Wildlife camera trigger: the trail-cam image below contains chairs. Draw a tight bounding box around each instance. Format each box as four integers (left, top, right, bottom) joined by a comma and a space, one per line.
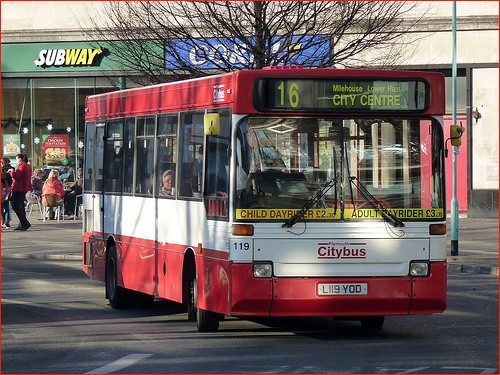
24, 193, 42, 221
41, 193, 65, 221
197, 156, 229, 194
73, 194, 83, 221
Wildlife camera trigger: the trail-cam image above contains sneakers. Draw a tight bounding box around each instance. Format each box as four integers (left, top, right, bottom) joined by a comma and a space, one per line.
44, 212, 77, 221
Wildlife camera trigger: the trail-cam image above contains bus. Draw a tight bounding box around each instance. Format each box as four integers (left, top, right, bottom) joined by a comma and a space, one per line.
81, 65, 465, 331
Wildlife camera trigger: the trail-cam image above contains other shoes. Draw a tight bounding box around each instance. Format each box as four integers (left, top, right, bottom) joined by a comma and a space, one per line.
22, 225, 31, 230
14, 225, 21, 231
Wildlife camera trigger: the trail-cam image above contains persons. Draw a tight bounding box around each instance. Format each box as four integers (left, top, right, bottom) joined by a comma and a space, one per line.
86, 110, 315, 225
29, 169, 55, 219
6, 154, 32, 231
63, 169, 82, 219
42, 170, 64, 219
1, 164, 12, 228
1, 158, 15, 229
